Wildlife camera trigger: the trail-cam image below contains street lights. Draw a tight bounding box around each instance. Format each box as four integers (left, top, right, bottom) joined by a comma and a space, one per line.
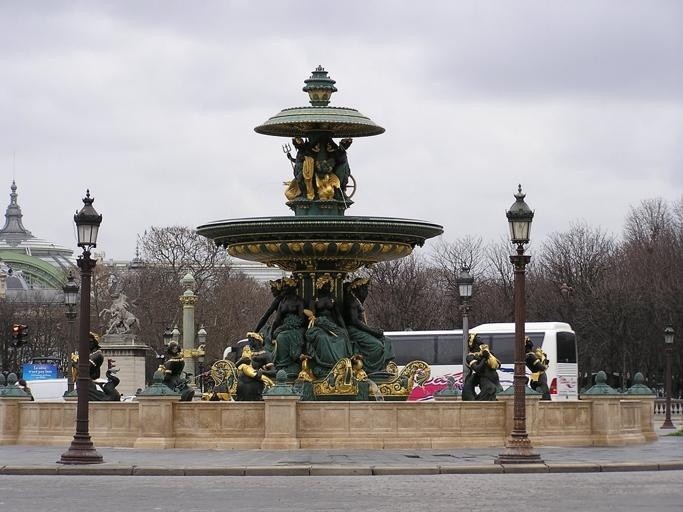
659, 322, 679, 429
198, 324, 206, 397
161, 323, 182, 352
456, 262, 475, 388
495, 185, 547, 463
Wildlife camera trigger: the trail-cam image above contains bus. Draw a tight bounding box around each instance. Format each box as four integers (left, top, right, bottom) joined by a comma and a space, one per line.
380, 322, 578, 400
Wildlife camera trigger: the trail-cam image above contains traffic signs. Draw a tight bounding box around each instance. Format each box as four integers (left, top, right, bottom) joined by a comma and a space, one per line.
56, 189, 105, 464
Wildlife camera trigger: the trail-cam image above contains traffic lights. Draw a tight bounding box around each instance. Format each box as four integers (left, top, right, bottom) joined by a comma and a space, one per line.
12, 323, 27, 348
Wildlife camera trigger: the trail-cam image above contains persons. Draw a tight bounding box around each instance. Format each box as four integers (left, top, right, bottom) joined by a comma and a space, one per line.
524, 335, 553, 400
283, 135, 311, 200
302, 137, 341, 201
159, 341, 185, 389
246, 273, 395, 379
464, 334, 502, 400
231, 331, 276, 400
331, 137, 352, 202
66, 331, 104, 381
